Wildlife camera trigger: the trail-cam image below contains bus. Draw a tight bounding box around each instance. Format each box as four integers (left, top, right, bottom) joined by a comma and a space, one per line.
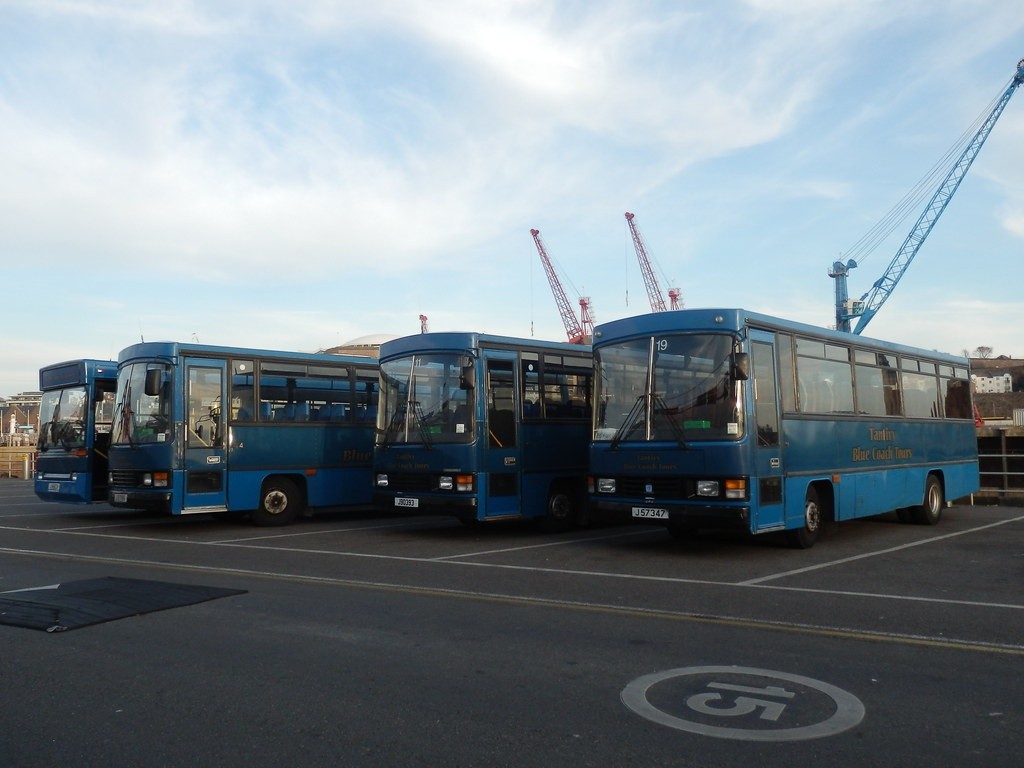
583, 307, 980, 550
370, 332, 770, 533
34, 359, 221, 506
106, 342, 465, 529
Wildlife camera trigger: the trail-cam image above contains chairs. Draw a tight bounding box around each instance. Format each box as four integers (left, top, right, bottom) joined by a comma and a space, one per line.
454, 396, 592, 420
784, 380, 945, 414
237, 401, 378, 423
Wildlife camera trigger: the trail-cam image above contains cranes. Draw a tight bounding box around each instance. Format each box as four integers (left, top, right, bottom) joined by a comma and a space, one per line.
825, 57, 1024, 338
623, 212, 685, 313
529, 228, 597, 347
